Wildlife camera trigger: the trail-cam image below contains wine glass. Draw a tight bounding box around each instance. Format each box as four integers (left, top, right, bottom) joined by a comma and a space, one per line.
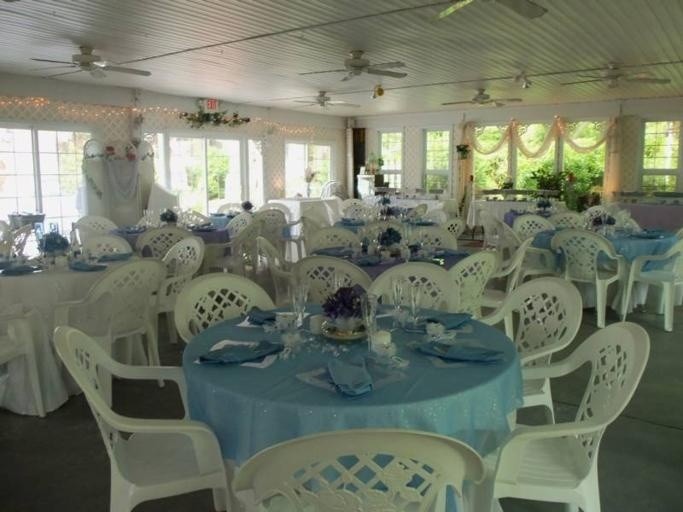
353, 192, 425, 263
389, 274, 424, 330
31, 221, 62, 263
575, 205, 633, 239
357, 292, 381, 357
287, 279, 311, 332
140, 204, 195, 232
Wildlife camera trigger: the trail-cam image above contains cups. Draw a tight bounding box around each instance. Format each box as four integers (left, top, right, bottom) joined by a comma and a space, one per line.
310, 314, 328, 335
376, 330, 392, 345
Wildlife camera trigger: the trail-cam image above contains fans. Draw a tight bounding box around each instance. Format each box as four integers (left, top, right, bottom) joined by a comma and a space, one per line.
559, 61, 671, 88
440, 88, 523, 108
30, 47, 151, 79
431, 0, 548, 24
298, 51, 408, 81
294, 91, 361, 111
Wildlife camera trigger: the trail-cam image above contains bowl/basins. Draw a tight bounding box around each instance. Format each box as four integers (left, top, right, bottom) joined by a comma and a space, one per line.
7, 213, 46, 232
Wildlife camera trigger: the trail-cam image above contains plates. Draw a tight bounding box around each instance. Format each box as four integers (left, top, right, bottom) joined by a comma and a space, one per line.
320, 318, 367, 341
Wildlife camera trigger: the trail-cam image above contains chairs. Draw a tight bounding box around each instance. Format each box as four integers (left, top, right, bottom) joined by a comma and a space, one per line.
483, 321, 651, 512
230, 428, 487, 512
1, 215, 133, 420
170, 250, 586, 428
298, 199, 468, 263
478, 200, 680, 332
52, 326, 228, 512
135, 205, 293, 305
54, 256, 170, 391
